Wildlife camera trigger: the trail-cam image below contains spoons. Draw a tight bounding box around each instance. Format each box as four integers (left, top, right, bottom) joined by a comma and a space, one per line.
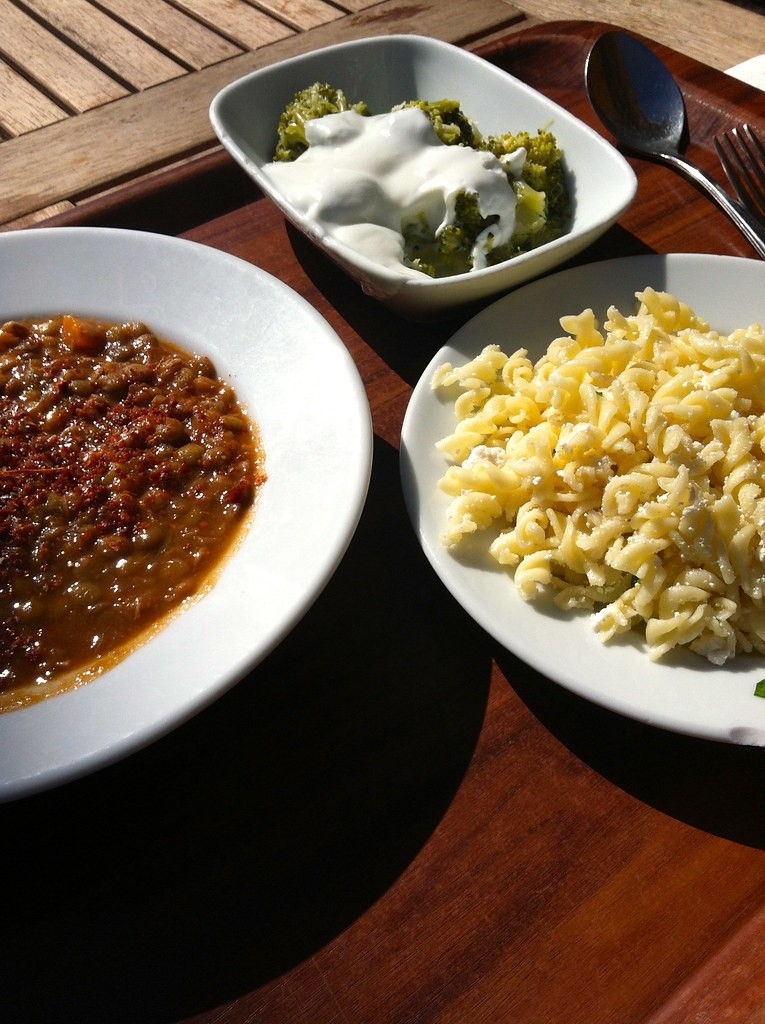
586, 30, 765, 264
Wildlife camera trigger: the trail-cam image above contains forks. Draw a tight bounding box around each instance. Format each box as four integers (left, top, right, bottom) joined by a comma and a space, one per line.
709, 122, 765, 239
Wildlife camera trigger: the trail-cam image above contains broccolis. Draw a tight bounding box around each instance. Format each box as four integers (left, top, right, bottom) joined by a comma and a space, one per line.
273, 81, 567, 277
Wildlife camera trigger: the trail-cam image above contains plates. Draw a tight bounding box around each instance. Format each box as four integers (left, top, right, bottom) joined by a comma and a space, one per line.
209, 35, 638, 318
0, 228, 374, 794
396, 253, 765, 747
